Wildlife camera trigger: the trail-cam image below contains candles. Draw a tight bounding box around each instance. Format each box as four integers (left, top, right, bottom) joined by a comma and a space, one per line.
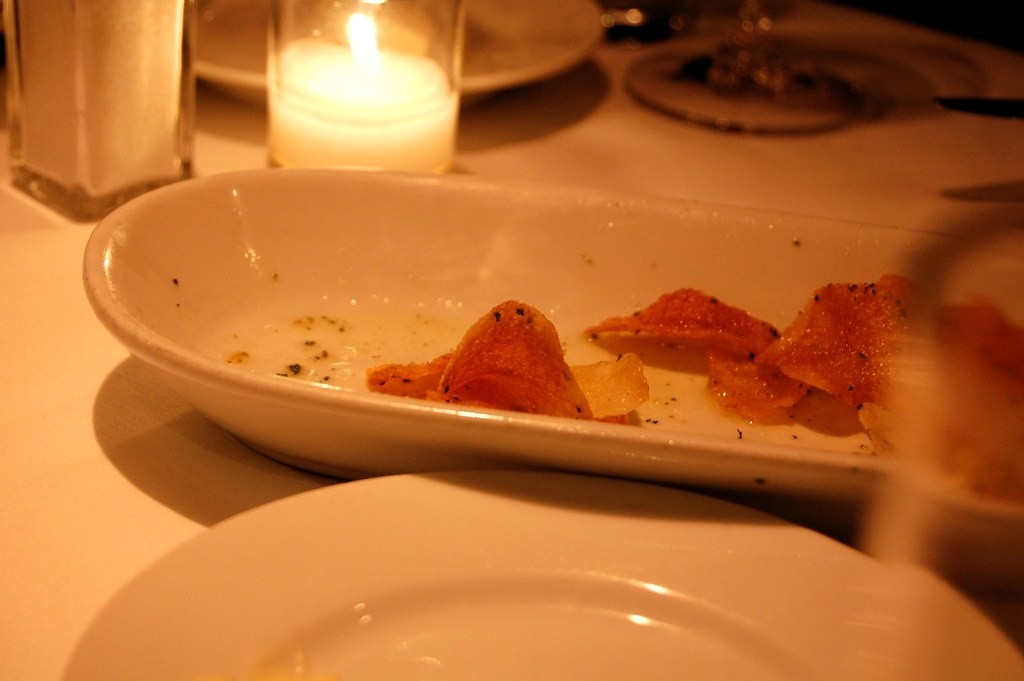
267, 11, 451, 174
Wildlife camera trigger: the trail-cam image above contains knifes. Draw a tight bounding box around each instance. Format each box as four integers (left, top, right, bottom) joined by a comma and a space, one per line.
932, 96, 1024, 120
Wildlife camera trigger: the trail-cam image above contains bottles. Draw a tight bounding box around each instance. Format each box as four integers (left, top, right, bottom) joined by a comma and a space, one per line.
0, 1, 194, 222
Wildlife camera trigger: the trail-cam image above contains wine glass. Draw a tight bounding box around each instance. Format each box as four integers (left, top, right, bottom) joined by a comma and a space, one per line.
628, 0, 853, 133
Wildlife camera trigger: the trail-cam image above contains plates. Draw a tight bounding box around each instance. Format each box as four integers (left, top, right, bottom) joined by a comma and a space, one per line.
59, 473, 1024, 680
84, 170, 1024, 506
186, 1, 604, 95
